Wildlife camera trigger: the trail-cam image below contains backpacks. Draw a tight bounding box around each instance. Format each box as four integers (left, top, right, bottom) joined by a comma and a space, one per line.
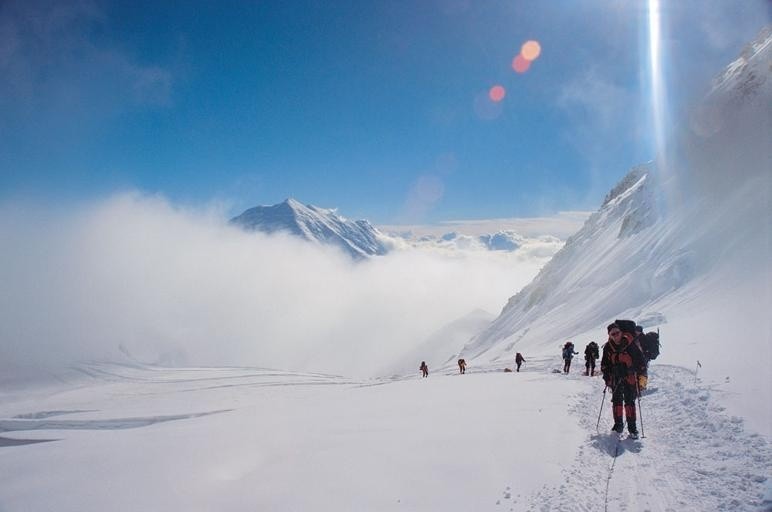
615, 319, 646, 375
644, 331, 659, 359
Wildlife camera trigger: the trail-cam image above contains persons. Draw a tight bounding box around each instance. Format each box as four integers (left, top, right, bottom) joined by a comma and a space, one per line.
516, 353, 525, 372
633, 326, 650, 397
421, 362, 428, 377
601, 324, 646, 438
458, 359, 466, 374
585, 342, 600, 376
563, 342, 579, 373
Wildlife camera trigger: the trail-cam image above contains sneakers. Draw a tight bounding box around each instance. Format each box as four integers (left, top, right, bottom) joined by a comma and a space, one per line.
628, 424, 638, 433
615, 423, 623, 433
